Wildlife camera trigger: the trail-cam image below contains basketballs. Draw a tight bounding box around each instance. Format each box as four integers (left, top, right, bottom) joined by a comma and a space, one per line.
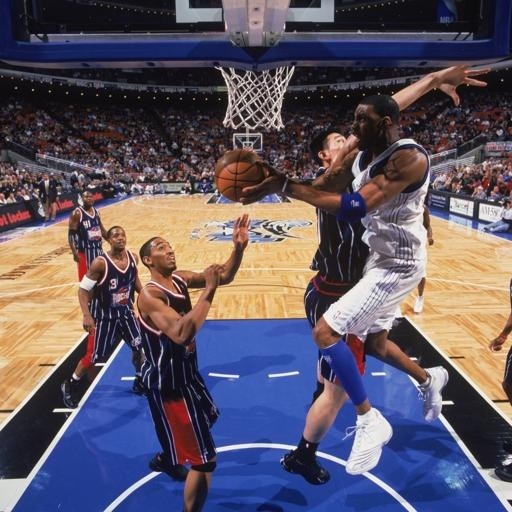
214, 150, 268, 202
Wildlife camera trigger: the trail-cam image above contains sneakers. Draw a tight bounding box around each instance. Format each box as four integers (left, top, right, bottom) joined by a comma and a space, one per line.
281, 450, 330, 485
414, 297, 424, 313
419, 366, 449, 421
495, 463, 512, 482
61, 379, 79, 408
132, 377, 147, 396
346, 409, 394, 476
150, 453, 189, 480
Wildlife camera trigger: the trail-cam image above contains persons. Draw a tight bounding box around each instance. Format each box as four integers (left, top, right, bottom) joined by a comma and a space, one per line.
489, 278, 512, 482
61, 225, 148, 407
239, 97, 450, 475
281, 63, 491, 485
2, 1, 512, 232
136, 213, 251, 511
68, 192, 110, 310
414, 202, 435, 313
